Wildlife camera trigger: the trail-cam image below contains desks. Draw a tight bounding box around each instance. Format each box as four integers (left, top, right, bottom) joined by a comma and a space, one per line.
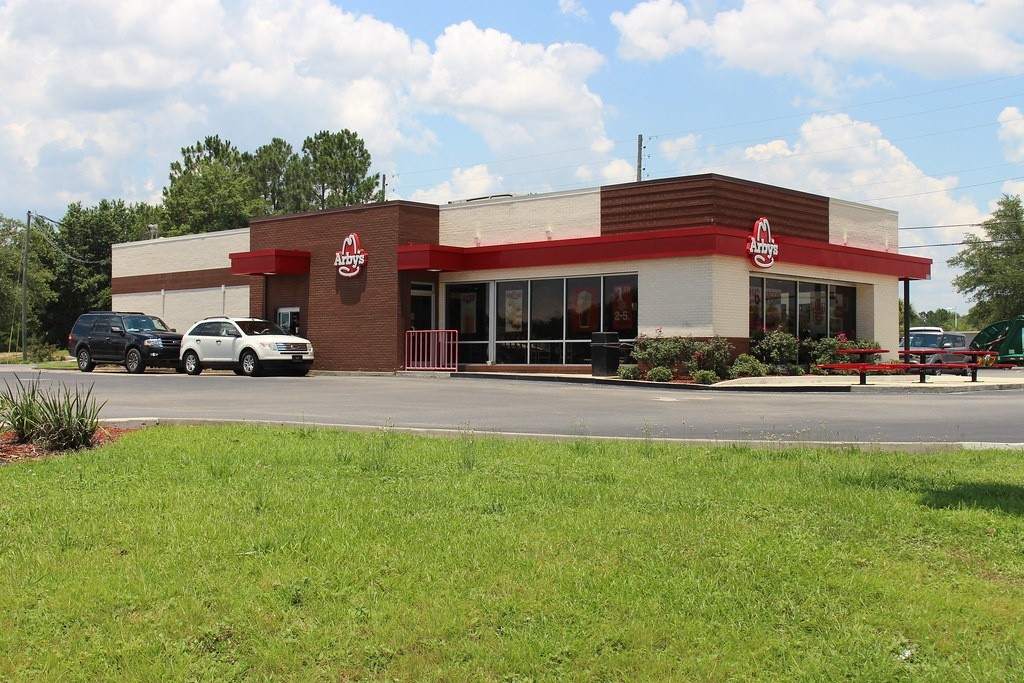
952, 351, 999, 382
896, 349, 946, 383
836, 349, 890, 385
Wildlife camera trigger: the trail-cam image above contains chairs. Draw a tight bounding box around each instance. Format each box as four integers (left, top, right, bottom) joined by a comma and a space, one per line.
524, 337, 576, 364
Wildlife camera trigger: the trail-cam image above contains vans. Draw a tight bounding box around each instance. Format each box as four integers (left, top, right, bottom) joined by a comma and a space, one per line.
899, 327, 945, 348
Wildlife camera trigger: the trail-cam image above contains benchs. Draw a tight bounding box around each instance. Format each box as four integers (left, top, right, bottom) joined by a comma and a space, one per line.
817, 364, 1015, 380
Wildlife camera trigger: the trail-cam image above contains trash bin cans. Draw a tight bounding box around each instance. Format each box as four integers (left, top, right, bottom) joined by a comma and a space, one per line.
590, 332, 620, 377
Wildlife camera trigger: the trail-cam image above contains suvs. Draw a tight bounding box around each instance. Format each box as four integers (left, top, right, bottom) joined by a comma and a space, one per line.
900, 332, 972, 377
178, 316, 315, 376
68, 311, 184, 374
